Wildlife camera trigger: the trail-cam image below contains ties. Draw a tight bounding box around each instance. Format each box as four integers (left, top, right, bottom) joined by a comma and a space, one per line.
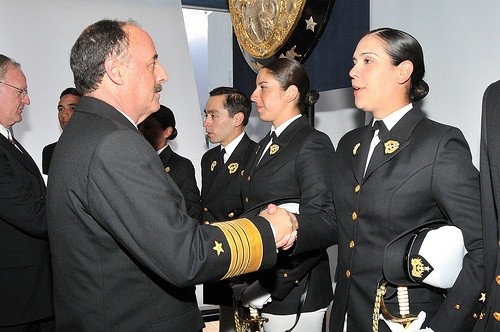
218, 148, 226, 169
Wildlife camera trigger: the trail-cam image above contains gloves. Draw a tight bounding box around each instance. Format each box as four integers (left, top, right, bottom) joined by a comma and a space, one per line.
233, 283, 272, 306
241, 279, 270, 310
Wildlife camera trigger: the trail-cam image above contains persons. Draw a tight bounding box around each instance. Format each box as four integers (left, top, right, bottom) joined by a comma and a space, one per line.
141, 103, 203, 227
41, 87, 82, 175
199, 55, 338, 332
259, 27, 500, 332
43, 18, 297, 332
0, 51, 56, 332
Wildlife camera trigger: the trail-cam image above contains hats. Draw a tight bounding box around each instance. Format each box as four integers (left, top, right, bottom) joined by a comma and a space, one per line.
238, 196, 301, 219
382, 219, 468, 288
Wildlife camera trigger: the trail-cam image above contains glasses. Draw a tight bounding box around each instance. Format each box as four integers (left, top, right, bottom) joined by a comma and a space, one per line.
0, 81, 28, 99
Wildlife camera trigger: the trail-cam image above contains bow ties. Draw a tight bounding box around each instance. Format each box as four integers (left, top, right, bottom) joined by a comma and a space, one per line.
270, 131, 278, 143
370, 120, 389, 140
